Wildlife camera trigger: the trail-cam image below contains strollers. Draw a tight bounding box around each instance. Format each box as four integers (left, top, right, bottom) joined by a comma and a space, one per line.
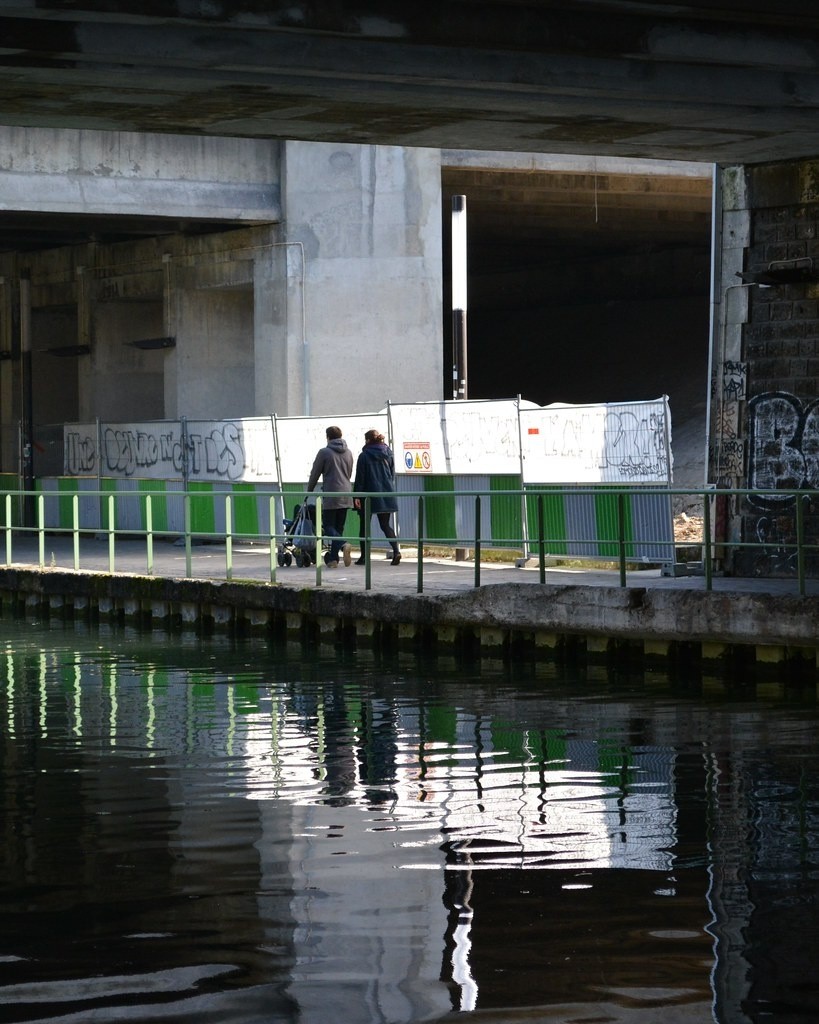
275, 496, 341, 569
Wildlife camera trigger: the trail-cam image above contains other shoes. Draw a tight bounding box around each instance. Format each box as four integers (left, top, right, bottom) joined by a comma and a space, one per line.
326, 561, 338, 568
341, 543, 351, 567
390, 553, 402, 566
355, 557, 365, 565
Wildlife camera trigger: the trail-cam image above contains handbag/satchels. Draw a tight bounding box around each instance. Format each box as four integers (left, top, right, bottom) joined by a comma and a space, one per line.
292, 501, 316, 551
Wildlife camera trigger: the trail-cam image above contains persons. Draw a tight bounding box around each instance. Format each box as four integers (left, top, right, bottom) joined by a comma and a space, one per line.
307, 426, 353, 568
352, 429, 402, 565
321, 671, 399, 808
